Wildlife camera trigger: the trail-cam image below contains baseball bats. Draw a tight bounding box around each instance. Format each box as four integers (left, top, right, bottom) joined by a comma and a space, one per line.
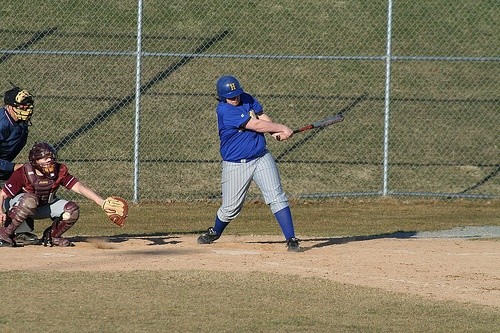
276, 114, 345, 141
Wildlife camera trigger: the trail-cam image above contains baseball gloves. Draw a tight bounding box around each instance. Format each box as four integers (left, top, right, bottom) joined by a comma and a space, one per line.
102, 195, 129, 227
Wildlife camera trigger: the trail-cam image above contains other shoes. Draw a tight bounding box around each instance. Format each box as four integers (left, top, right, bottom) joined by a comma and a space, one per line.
50, 237, 70, 246
8, 236, 24, 247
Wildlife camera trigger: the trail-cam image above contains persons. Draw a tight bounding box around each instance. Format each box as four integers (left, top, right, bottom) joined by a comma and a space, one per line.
197, 73, 300, 253
0, 143, 128, 247
0, 86, 34, 190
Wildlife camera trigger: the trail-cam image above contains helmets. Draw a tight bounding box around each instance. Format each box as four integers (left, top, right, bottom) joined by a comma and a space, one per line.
4, 88, 34, 109
217, 75, 243, 99
28, 142, 58, 180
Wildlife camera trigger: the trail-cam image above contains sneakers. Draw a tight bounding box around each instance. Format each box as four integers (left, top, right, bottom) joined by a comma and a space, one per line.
285, 237, 301, 252
197, 227, 221, 244
